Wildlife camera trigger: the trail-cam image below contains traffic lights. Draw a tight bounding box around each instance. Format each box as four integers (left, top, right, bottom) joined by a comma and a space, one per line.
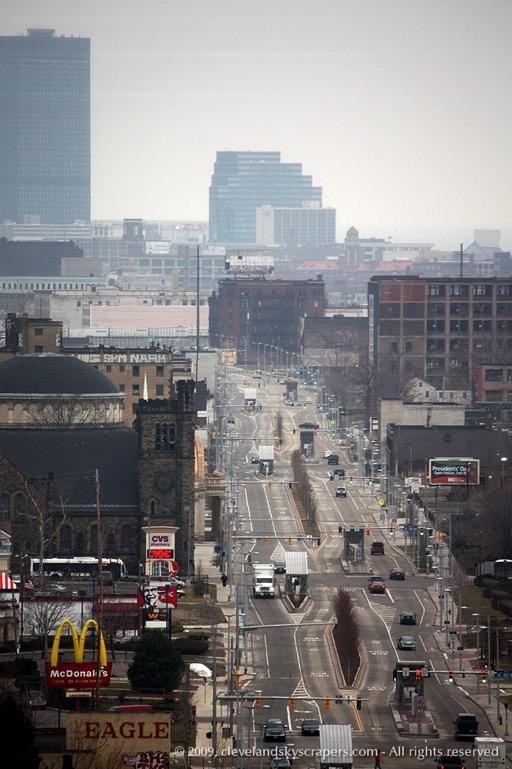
416, 669, 421, 681
393, 669, 397, 682
338, 527, 342, 536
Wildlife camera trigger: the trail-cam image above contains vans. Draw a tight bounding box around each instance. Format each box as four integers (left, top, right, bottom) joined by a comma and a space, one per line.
453, 712, 479, 741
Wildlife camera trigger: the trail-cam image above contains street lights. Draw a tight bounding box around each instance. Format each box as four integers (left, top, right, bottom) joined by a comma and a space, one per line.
363, 440, 421, 571
427, 535, 502, 720
309, 360, 352, 454
208, 376, 271, 769
215, 333, 306, 379
15, 552, 28, 652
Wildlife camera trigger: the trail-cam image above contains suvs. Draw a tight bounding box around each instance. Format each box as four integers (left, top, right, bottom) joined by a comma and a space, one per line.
28, 691, 47, 710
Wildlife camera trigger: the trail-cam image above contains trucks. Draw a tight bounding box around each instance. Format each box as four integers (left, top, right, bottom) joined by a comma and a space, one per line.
472, 737, 507, 768
320, 724, 353, 769
253, 563, 275, 599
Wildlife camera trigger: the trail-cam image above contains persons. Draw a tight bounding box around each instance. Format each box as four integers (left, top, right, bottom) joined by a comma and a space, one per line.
374, 748, 382, 769
137, 585, 177, 610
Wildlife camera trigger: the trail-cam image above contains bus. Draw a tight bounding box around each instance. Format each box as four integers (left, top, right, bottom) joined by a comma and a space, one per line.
30, 556, 127, 579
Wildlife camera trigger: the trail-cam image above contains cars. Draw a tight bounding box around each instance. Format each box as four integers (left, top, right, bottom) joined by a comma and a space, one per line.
270, 743, 295, 769
434, 756, 466, 769
368, 576, 386, 594
324, 450, 354, 498
389, 567, 405, 580
262, 719, 288, 742
140, 576, 186, 598
274, 561, 286, 573
399, 611, 417, 625
397, 635, 416, 650
302, 719, 321, 736
